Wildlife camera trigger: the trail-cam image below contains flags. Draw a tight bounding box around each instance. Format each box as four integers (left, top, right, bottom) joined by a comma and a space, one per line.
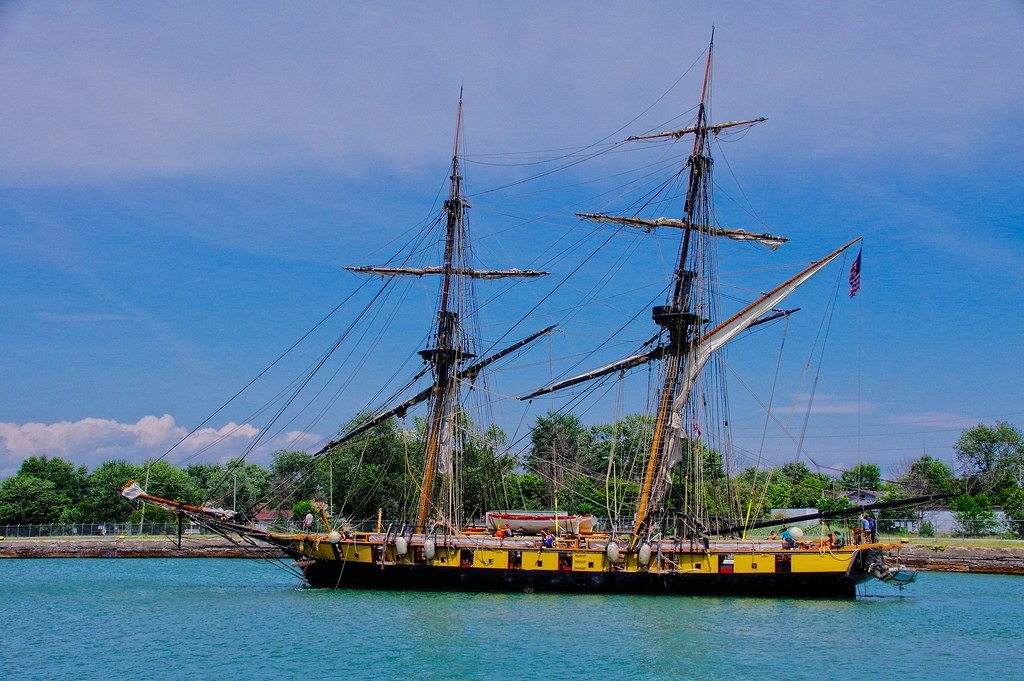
848, 249, 862, 298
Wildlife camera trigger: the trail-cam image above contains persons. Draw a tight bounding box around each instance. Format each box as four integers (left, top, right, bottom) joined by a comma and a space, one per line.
303, 510, 313, 532
767, 530, 779, 540
864, 509, 876, 543
780, 527, 796, 547
823, 534, 835, 545
834, 531, 843, 545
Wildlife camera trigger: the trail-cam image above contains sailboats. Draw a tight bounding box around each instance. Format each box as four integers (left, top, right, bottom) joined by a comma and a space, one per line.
118, 27, 917, 599
